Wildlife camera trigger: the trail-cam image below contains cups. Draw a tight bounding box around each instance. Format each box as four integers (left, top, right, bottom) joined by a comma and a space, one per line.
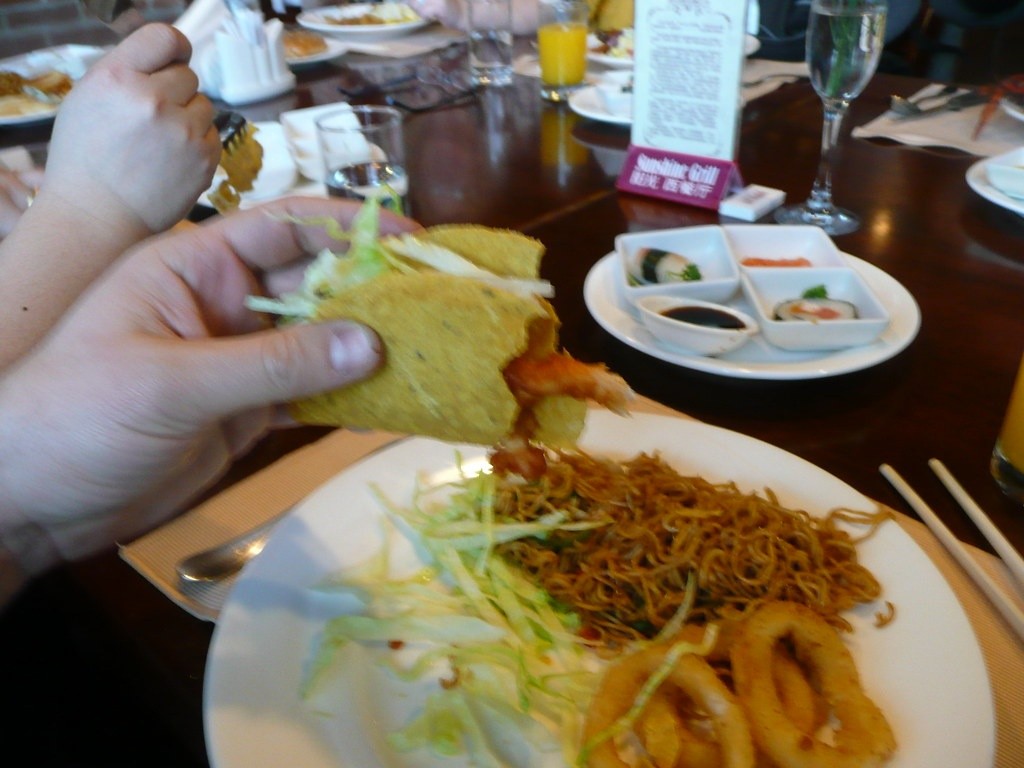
466, 1, 515, 89
537, 0, 588, 102
315, 104, 412, 219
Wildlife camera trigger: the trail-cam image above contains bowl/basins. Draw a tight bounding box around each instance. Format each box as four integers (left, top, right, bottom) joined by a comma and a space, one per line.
983, 147, 1024, 202
616, 221, 892, 355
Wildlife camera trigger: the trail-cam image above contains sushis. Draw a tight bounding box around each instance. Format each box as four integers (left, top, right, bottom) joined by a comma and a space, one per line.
631, 247, 700, 281
774, 296, 858, 320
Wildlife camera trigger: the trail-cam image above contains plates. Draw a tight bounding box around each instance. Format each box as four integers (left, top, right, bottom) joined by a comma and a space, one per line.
964, 154, 1024, 217
566, 85, 630, 126
586, 30, 761, 70
195, 120, 390, 208
284, 37, 349, 69
296, 2, 430, 44
584, 249, 920, 380
202, 406, 996, 768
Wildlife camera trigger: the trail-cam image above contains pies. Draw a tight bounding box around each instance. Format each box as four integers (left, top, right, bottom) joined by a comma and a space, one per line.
207, 119, 264, 213
245, 167, 636, 452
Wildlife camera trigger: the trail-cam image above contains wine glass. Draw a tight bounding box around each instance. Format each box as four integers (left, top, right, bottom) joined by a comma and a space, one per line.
774, 0, 888, 240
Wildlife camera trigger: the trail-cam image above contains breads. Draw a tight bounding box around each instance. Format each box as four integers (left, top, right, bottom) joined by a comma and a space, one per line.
284, 31, 326, 57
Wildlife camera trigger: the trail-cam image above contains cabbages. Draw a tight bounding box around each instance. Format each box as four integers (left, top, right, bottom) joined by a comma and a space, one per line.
298, 454, 718, 768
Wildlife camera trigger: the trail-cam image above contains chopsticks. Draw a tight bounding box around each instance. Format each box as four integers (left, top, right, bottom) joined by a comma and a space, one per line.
878, 459, 1024, 643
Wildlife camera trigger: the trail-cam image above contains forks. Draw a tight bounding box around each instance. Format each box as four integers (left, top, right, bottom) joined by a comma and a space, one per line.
211, 109, 249, 155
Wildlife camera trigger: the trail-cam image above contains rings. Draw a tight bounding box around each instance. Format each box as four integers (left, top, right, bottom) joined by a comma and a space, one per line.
417, 0, 425, 6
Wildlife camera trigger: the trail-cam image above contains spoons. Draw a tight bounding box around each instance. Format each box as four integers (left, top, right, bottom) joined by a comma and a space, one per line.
881, 88, 986, 117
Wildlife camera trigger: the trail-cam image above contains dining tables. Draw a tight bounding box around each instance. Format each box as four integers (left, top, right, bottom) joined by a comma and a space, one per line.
0, 0, 1023, 767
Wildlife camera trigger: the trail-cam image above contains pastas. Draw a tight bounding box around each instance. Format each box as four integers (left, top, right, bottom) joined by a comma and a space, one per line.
475, 450, 896, 651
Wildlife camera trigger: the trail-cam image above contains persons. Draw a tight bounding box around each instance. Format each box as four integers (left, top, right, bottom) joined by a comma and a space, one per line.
385, 0, 636, 44
0, 196, 430, 601
0, 23, 223, 376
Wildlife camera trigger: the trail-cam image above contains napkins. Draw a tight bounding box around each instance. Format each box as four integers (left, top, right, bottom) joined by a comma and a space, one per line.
852, 84, 1023, 158
738, 59, 810, 104
